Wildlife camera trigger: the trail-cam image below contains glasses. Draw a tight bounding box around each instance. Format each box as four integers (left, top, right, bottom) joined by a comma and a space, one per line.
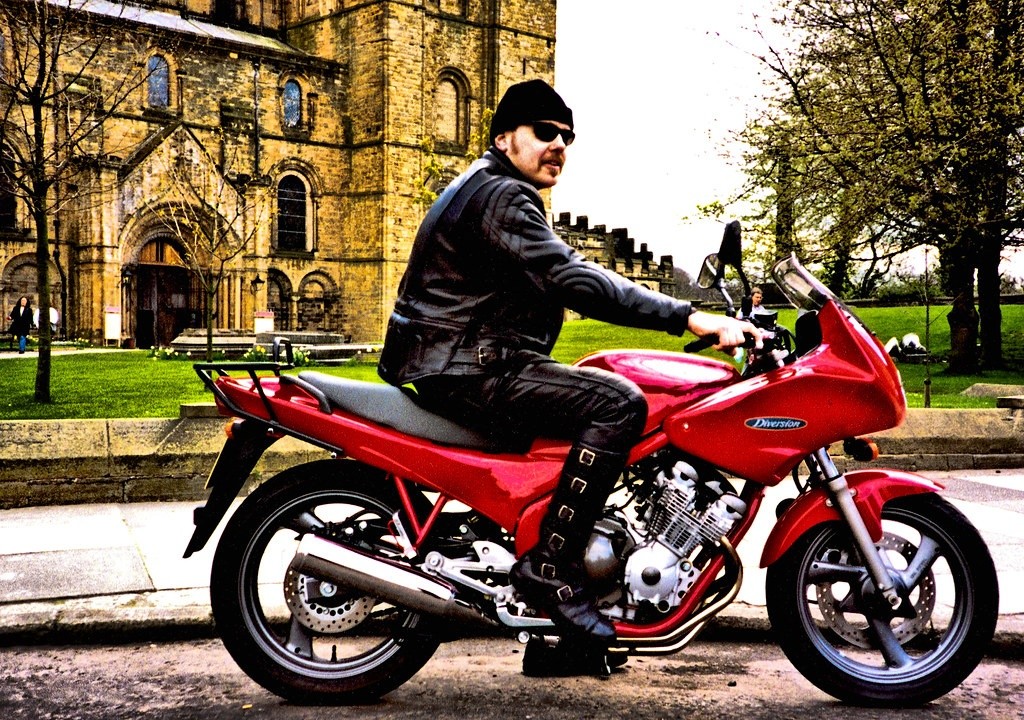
524, 118, 576, 146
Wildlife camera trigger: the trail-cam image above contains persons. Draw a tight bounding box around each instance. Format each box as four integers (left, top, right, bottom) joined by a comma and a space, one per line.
737, 287, 766, 320
376, 80, 765, 677
7, 297, 34, 354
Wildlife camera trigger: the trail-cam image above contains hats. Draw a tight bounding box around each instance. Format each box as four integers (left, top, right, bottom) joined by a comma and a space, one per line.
489, 79, 573, 142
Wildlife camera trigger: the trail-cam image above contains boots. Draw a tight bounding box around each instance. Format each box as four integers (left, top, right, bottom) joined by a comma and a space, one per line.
519, 630, 630, 677
509, 440, 633, 646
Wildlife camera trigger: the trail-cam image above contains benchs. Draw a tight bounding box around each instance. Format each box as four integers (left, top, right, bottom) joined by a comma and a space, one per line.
997, 395, 1024, 421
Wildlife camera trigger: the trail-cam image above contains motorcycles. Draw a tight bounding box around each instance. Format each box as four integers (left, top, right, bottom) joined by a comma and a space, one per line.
183, 220, 1000, 712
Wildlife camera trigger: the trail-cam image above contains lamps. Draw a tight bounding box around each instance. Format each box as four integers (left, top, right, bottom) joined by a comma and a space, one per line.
108, 155, 123, 164
249, 273, 265, 297
236, 173, 251, 184
116, 265, 133, 288
